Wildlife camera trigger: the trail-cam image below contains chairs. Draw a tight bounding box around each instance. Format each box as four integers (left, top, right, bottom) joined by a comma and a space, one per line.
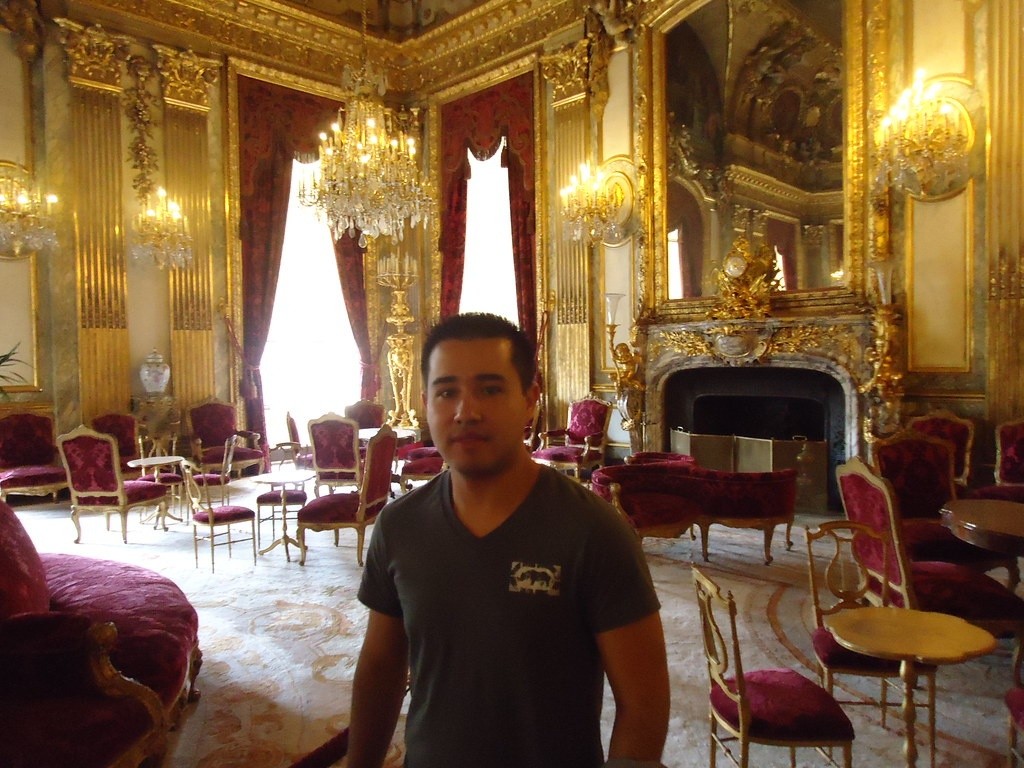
0, 396, 451, 574
522, 393, 613, 485
689, 410, 1024, 768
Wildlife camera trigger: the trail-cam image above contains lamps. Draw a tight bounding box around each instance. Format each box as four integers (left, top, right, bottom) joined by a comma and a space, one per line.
129, 185, 194, 270
0, 159, 63, 261
874, 69, 984, 201
296, 0, 435, 248
560, 156, 643, 248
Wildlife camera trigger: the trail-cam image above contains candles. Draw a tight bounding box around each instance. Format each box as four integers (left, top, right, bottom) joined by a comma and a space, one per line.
376, 246, 418, 277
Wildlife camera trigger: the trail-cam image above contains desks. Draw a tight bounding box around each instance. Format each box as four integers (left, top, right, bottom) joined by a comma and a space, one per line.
357, 428, 417, 475
248, 470, 316, 562
939, 498, 1024, 557
821, 607, 995, 768
126, 456, 185, 533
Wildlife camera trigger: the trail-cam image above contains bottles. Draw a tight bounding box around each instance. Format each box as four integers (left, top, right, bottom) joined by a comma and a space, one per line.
140, 348, 171, 399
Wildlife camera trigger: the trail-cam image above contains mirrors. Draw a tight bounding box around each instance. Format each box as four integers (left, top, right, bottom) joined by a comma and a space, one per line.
644, 0, 862, 323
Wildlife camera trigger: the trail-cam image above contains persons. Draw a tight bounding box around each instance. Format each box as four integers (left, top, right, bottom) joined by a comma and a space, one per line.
347, 313, 672, 767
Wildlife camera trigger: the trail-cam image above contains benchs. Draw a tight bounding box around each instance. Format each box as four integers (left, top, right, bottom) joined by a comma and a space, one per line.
0, 500, 203, 768
591, 465, 798, 567
